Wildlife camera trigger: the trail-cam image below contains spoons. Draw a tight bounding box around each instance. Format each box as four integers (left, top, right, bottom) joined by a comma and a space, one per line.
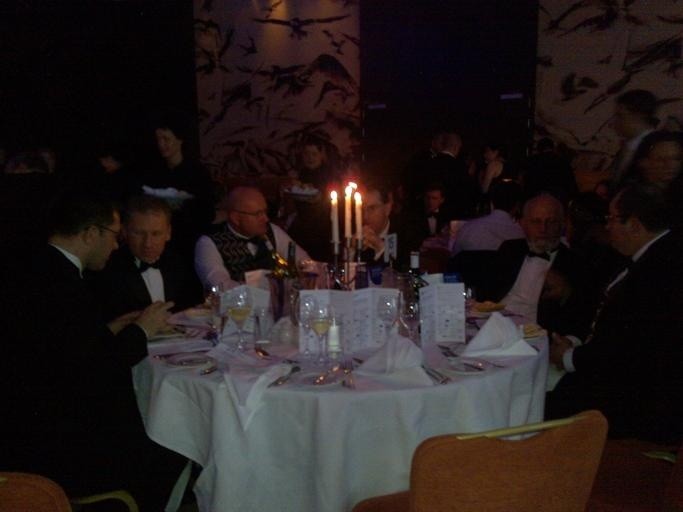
273, 364, 300, 386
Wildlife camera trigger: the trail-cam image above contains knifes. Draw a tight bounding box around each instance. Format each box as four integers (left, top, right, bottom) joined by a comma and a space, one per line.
152, 347, 211, 359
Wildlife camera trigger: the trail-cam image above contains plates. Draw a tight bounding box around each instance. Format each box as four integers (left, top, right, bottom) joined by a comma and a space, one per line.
145, 194, 190, 208
280, 191, 317, 202
182, 309, 211, 320
288, 367, 344, 389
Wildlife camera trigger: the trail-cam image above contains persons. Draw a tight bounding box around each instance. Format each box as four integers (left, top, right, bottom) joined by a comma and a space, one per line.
344, 172, 416, 274
398, 87, 681, 443
134, 122, 216, 215
0, 188, 191, 510
98, 193, 206, 329
193, 181, 316, 298
274, 137, 334, 250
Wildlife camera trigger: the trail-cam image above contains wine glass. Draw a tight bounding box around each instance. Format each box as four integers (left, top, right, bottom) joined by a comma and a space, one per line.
377, 295, 420, 346
223, 287, 251, 350
294, 295, 331, 367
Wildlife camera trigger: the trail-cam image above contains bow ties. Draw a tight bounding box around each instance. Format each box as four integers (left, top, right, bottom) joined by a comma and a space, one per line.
139, 261, 159, 271
528, 251, 550, 261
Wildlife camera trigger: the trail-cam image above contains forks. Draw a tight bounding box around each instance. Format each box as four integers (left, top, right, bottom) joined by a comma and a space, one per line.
344, 359, 354, 391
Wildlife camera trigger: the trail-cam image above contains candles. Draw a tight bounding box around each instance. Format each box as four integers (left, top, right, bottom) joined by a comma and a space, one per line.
344, 185, 352, 238
329, 190, 339, 242
355, 195, 362, 239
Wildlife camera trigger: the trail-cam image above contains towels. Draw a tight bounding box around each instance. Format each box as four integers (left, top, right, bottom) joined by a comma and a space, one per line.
461, 309, 540, 358
223, 361, 293, 432
355, 332, 434, 386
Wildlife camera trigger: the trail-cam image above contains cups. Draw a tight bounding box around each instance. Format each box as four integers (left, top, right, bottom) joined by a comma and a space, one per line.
252, 304, 273, 358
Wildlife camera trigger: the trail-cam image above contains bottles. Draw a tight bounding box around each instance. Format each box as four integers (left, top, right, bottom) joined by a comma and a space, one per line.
405, 251, 422, 321
257, 234, 300, 324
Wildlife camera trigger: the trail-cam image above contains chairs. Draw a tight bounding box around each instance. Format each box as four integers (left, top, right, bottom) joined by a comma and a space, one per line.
0, 471, 139, 512
349, 409, 609, 512
